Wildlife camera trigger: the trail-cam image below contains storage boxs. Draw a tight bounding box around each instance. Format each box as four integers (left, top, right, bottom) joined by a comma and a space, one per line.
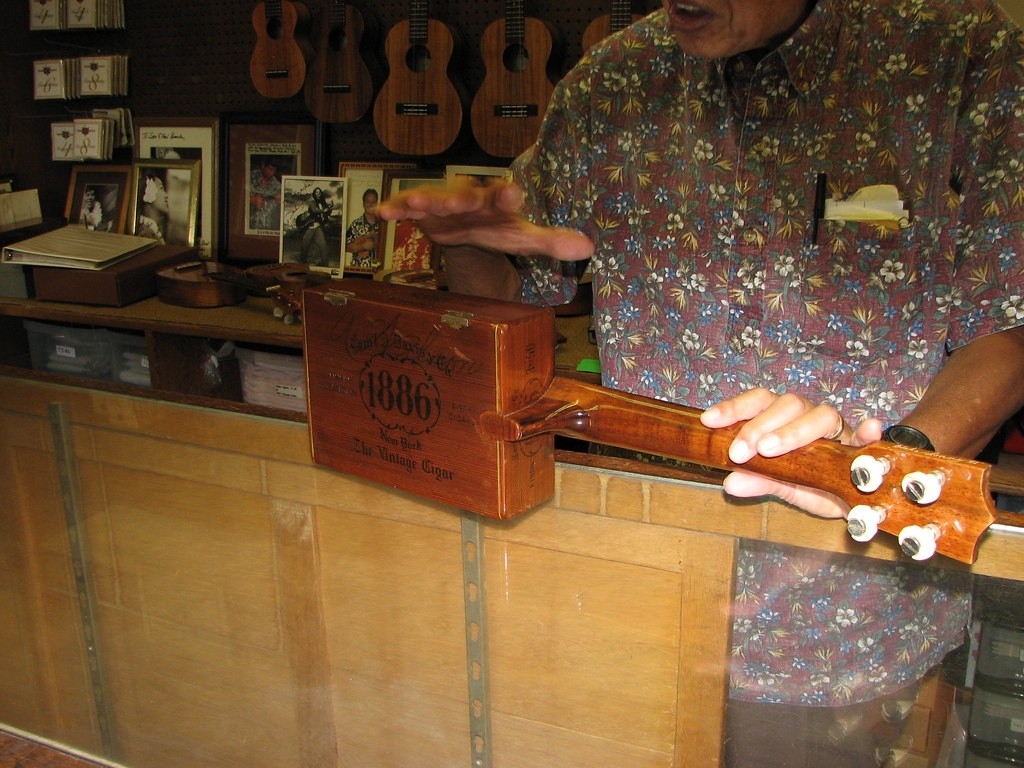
237, 349, 309, 412
21, 318, 110, 378
34, 243, 192, 306
110, 333, 152, 386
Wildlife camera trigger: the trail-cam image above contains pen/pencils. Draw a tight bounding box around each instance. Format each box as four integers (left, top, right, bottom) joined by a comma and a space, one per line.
812, 171, 825, 244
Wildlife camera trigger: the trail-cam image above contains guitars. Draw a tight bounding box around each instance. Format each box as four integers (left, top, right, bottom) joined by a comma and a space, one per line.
297, 275, 1001, 569
249, 1, 651, 160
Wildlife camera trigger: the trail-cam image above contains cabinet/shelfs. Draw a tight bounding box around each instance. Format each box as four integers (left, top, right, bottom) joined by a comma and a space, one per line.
0, 299, 1024, 526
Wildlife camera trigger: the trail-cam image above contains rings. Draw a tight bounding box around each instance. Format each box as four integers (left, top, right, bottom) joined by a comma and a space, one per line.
828, 412, 844, 441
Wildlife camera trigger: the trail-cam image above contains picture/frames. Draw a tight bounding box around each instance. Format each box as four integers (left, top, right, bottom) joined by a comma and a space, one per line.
337, 161, 417, 276
279, 175, 348, 279
63, 165, 133, 235
131, 114, 221, 263
375, 169, 445, 282
128, 157, 203, 248
218, 110, 325, 263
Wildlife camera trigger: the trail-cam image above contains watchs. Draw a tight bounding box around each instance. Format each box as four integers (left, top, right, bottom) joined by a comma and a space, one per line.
882, 424, 935, 452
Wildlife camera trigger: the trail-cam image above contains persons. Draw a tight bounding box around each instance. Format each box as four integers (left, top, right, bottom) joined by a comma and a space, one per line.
345, 189, 380, 268
250, 158, 283, 230
136, 174, 172, 244
369, 0, 1024, 768
298, 187, 336, 267
79, 184, 105, 232
